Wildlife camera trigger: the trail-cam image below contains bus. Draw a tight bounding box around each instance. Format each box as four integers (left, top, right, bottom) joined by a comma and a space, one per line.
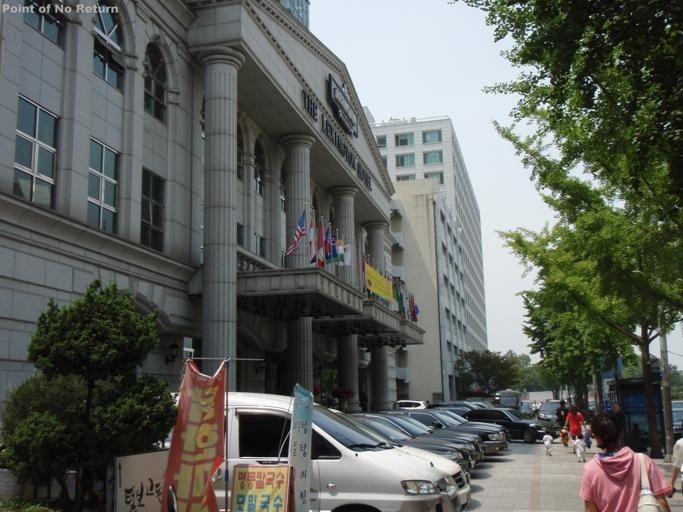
606, 378, 664, 439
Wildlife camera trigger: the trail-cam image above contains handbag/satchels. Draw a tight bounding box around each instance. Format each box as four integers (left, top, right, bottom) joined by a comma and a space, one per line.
637, 490, 664, 512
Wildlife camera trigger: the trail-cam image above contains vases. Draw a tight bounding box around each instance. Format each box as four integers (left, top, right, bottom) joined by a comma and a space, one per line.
341, 397, 348, 408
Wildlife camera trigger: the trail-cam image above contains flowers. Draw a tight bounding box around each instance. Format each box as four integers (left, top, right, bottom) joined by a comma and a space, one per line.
331, 386, 354, 399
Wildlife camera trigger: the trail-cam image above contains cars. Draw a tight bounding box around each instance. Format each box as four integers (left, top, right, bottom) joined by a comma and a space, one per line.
152, 390, 560, 512
671, 400, 683, 437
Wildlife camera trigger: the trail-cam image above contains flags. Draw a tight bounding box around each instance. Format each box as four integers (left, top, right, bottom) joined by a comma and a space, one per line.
285, 211, 419, 322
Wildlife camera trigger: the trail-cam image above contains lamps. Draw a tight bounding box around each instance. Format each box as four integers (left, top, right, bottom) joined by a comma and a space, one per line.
164, 339, 179, 364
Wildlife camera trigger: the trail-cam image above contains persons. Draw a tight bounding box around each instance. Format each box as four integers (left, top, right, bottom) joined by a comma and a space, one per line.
360, 383, 368, 413
529, 397, 647, 463
579, 411, 675, 511
667, 437, 683, 499
